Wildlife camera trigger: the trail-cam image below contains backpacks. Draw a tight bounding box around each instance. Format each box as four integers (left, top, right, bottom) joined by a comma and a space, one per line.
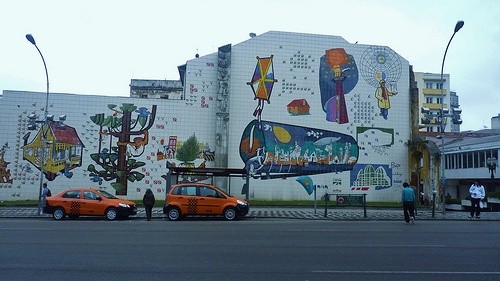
47, 189, 51, 196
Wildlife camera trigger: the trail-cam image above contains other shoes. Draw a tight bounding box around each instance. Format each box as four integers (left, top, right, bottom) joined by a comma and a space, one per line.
476, 216, 480, 218
468, 215, 473, 218
410, 216, 415, 225
403, 222, 409, 225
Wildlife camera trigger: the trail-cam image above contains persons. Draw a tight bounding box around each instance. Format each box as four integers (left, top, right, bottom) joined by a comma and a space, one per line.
143, 189, 155, 221
402, 182, 415, 224
469, 179, 485, 218
41, 183, 48, 203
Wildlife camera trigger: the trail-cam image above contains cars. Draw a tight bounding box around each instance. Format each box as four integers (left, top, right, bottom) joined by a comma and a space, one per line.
163, 182, 250, 221
42, 186, 138, 222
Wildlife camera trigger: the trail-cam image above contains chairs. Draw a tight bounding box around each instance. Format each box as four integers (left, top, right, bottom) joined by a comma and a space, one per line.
87, 193, 93, 199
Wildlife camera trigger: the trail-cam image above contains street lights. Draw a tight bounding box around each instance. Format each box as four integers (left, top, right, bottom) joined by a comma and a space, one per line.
24, 32, 68, 216
420, 19, 465, 215
486, 157, 496, 192
